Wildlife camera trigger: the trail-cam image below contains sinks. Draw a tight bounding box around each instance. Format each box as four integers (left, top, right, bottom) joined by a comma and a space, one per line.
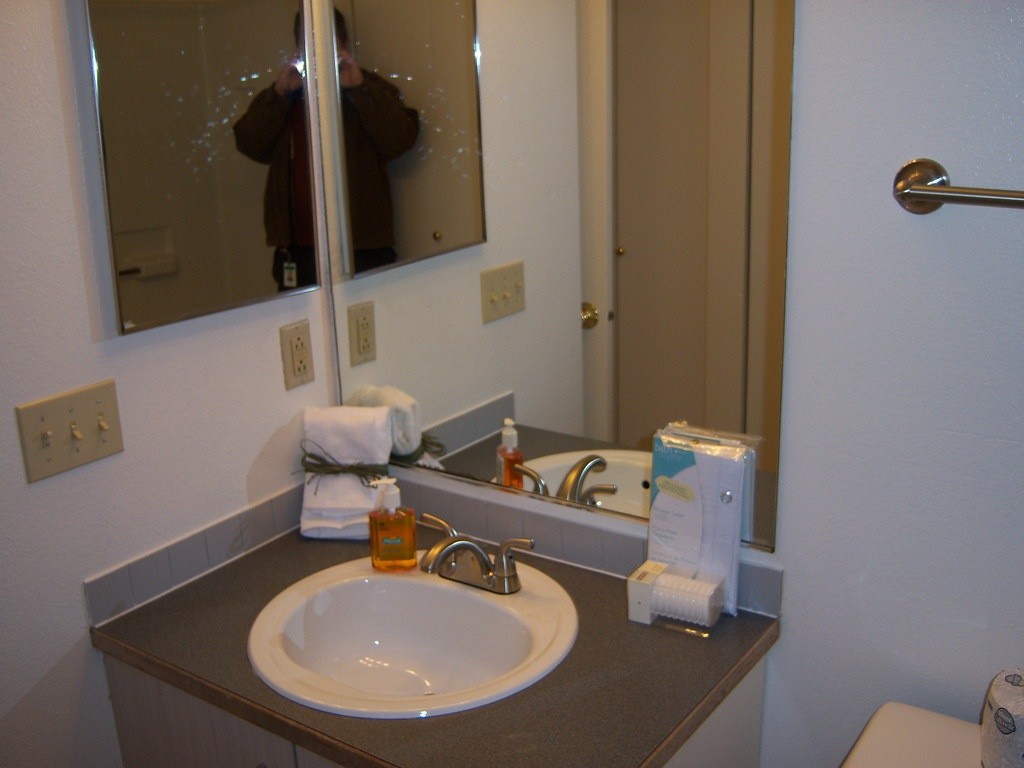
246, 545, 576, 721
488, 448, 653, 527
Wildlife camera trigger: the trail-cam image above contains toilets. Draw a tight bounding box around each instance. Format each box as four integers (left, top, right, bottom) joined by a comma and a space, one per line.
841, 698, 980, 768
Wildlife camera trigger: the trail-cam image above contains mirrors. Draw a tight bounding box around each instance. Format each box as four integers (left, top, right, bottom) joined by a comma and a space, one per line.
86, 0, 321, 335
327, 0, 798, 554
326, 0, 486, 281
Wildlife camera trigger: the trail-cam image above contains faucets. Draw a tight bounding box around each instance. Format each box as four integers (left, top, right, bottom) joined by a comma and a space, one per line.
404, 508, 536, 598
498, 447, 617, 509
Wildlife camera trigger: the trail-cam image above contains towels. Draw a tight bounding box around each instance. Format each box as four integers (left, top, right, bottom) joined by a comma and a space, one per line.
345, 385, 445, 471
298, 405, 394, 540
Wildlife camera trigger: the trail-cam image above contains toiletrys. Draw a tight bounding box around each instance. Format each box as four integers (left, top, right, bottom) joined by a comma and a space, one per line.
367, 478, 418, 574
496, 416, 524, 490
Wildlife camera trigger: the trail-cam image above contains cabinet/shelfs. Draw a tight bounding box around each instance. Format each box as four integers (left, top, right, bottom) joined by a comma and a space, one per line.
101, 650, 765, 768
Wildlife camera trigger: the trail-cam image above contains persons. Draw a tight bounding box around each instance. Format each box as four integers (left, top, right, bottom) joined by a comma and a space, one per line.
233, 4, 420, 293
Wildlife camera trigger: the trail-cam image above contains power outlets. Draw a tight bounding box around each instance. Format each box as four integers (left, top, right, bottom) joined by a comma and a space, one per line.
347, 300, 376, 368
279, 318, 316, 390
